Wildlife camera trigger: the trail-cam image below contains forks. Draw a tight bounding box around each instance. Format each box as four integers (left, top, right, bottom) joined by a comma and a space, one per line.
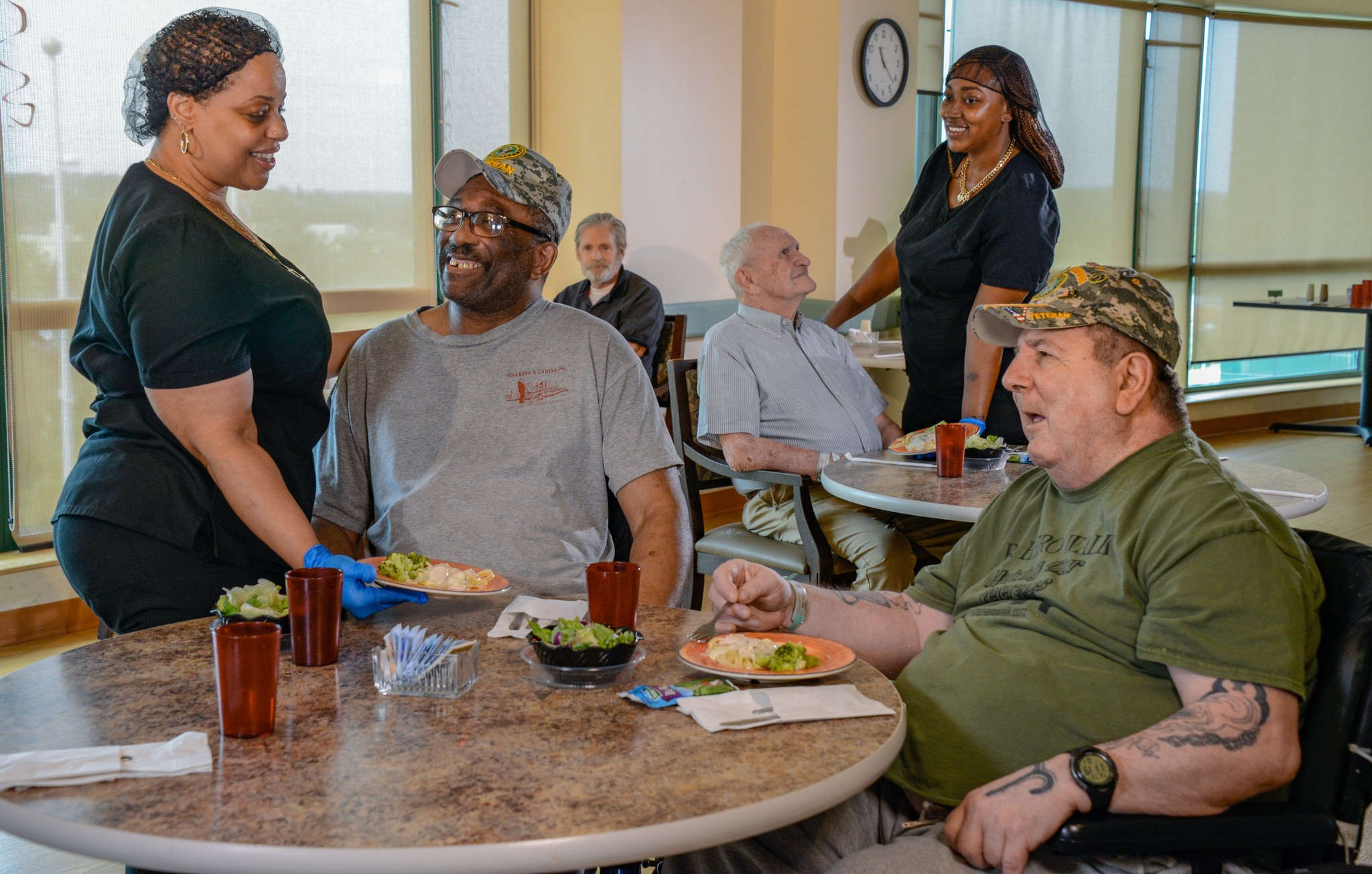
687, 600, 734, 643
1005, 445, 1024, 453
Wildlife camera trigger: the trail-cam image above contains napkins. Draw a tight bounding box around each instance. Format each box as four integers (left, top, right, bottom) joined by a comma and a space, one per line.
488, 595, 589, 638
0, 730, 213, 793
677, 683, 898, 736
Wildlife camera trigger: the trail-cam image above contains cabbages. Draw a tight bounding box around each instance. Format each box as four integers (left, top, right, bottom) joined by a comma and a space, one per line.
216, 579, 290, 619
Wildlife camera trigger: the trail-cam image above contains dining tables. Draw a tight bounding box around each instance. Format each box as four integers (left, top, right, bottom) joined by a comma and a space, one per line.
0, 592, 903, 874
820, 443, 1328, 524
834, 329, 905, 370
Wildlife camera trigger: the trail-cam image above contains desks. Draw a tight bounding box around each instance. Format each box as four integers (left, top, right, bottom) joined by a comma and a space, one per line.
1233, 296, 1372, 446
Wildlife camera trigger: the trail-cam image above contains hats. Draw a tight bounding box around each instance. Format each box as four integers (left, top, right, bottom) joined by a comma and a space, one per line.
970, 262, 1181, 370
433, 143, 572, 248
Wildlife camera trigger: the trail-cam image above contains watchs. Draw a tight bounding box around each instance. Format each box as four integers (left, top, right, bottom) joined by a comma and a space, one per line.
1062, 743, 1120, 820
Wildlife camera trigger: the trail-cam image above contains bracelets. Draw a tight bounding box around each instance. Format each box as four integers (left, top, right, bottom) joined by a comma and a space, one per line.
783, 579, 808, 631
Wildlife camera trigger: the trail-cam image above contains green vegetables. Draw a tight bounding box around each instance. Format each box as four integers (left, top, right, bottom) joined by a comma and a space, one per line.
526, 616, 636, 651
923, 420, 1006, 449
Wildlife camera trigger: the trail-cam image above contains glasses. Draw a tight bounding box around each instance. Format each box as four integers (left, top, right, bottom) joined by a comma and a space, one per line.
431, 205, 552, 243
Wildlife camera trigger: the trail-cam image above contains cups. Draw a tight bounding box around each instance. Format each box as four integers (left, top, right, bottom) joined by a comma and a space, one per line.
585, 561, 641, 631
284, 567, 344, 667
260, 652, 261, 653
1349, 284, 1362, 309
1306, 284, 1314, 301
935, 424, 967, 478
1362, 280, 1372, 308
1320, 284, 1328, 302
212, 622, 282, 740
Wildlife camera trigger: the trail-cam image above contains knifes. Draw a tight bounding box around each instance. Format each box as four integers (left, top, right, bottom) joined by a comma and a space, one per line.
718, 713, 780, 726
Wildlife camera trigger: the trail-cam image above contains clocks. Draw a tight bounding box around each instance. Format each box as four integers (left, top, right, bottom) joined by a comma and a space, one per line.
859, 18, 909, 107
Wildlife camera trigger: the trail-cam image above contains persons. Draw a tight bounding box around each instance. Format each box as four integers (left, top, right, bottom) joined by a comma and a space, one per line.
815, 40, 1065, 449
663, 261, 1329, 874
47, 0, 430, 642
290, 139, 692, 621
704, 225, 977, 604
548, 212, 665, 576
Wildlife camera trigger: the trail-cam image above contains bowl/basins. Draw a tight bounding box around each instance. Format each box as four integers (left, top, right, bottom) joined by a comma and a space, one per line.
371, 639, 478, 699
847, 331, 880, 345
210, 607, 292, 651
963, 443, 1009, 470
520, 622, 644, 685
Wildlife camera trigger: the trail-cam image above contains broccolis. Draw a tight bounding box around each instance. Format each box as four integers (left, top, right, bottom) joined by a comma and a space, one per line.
756, 642, 820, 671
378, 551, 430, 581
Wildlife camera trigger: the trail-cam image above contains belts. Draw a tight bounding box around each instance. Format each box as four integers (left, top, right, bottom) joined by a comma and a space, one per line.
746, 489, 761, 500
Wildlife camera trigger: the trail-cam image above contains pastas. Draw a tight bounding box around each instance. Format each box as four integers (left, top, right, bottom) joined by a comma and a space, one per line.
888, 431, 936, 450
408, 561, 493, 590
706, 637, 772, 669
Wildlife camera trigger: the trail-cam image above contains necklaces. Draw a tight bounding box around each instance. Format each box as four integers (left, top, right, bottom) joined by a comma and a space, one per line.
143, 155, 313, 285
956, 136, 1015, 204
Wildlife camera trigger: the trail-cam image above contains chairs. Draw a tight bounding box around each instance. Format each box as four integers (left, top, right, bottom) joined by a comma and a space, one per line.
648, 313, 1372, 874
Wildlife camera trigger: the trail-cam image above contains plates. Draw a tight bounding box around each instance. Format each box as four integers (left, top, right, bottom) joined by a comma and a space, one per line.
677, 632, 858, 683
888, 423, 980, 455
355, 556, 512, 600
